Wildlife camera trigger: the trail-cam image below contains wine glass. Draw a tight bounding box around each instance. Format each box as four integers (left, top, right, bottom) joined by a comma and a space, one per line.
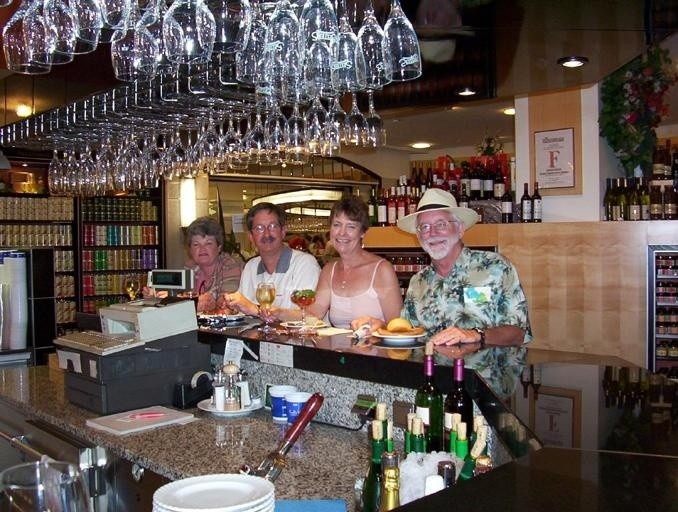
256, 283, 278, 331
0, 1, 424, 198
124, 278, 141, 300
292, 292, 316, 336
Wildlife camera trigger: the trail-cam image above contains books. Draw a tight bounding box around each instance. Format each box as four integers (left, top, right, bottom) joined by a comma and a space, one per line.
86, 405, 194, 436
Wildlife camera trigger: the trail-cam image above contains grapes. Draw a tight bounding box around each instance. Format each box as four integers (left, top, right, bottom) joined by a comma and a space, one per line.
293, 288, 317, 309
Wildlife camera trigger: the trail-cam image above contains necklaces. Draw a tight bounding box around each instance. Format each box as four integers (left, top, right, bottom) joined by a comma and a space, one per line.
341, 251, 364, 288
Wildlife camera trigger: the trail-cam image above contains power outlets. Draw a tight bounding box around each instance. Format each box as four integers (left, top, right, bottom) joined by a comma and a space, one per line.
350, 394, 377, 422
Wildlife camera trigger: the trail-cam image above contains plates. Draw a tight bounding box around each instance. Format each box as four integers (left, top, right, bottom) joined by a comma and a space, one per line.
280, 321, 332, 328
148, 471, 278, 512
196, 398, 266, 416
372, 331, 427, 342
198, 310, 246, 320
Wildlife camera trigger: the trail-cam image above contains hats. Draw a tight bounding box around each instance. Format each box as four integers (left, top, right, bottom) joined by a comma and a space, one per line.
394, 186, 480, 235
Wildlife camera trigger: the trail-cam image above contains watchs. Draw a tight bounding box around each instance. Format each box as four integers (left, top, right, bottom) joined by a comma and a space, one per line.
471, 328, 485, 343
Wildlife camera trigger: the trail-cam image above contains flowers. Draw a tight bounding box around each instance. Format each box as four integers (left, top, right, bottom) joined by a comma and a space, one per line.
600, 43, 678, 174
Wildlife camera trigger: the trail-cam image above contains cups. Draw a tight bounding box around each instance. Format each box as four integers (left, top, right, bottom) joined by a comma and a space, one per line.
0, 461, 93, 511
268, 384, 297, 422
0, 250, 28, 351
286, 393, 312, 429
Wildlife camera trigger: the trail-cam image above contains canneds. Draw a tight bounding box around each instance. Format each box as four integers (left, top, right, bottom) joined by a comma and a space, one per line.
82, 272, 149, 296
83, 297, 131, 314
81, 199, 158, 222
55, 275, 76, 297
54, 251, 74, 272
81, 249, 158, 270
81, 224, 157, 247
0, 223, 73, 247
54, 299, 76, 325
138, 188, 150, 198
2, 196, 73, 220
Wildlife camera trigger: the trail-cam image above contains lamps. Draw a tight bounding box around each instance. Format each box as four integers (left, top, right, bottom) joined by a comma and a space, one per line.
178, 169, 198, 235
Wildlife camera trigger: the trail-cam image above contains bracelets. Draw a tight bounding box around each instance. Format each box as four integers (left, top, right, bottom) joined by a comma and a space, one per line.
473, 343, 486, 354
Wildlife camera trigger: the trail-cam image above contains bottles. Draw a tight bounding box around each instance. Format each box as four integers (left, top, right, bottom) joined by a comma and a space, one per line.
356, 159, 678, 230
602, 365, 678, 432
213, 359, 255, 410
355, 356, 493, 509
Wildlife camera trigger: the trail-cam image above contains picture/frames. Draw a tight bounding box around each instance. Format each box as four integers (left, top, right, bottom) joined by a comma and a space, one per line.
534, 126, 576, 190
526, 384, 582, 447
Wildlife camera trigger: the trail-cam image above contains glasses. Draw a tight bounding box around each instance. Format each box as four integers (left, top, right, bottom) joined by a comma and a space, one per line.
414, 219, 459, 232
250, 223, 282, 234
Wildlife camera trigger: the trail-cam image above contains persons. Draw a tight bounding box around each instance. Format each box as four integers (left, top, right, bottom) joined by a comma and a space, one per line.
350, 188, 533, 346
432, 343, 528, 403
288, 231, 337, 256
259, 196, 403, 330
225, 202, 321, 323
142, 217, 240, 315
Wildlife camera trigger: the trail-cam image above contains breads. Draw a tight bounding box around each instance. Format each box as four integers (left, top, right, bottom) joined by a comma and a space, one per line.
377, 327, 425, 335
386, 319, 414, 331
